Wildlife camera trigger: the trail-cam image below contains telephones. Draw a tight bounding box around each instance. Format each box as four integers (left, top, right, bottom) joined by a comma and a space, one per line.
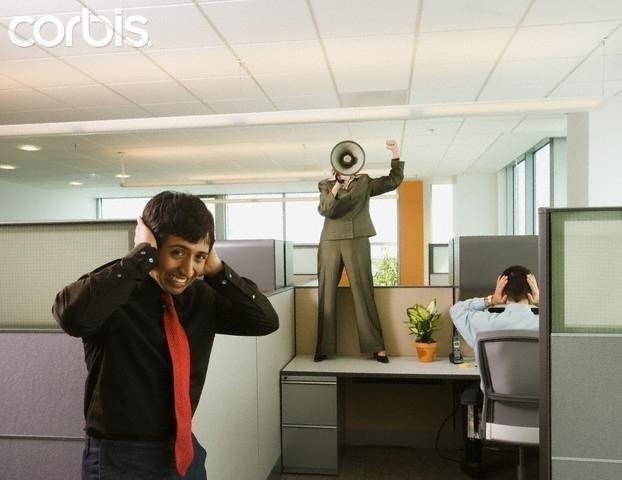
449, 329, 463, 364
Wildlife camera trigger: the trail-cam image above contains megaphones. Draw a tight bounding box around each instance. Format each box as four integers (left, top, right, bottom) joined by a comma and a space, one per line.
331, 140, 365, 175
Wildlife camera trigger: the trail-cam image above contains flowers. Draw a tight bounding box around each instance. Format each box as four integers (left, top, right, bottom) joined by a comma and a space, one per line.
402, 299, 444, 342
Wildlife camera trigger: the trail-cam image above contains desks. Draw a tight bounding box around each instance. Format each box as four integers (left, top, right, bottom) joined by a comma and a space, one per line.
279, 355, 479, 474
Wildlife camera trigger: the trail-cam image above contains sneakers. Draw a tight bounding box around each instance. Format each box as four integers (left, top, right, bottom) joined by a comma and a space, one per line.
460, 459, 481, 479
313, 354, 326, 362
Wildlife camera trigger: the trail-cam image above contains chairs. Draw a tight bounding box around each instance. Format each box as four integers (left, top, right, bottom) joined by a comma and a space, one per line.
460, 331, 540, 480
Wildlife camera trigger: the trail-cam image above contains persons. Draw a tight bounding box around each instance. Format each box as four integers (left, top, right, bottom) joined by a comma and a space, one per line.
448, 266, 540, 479
311, 137, 406, 365
50, 188, 282, 480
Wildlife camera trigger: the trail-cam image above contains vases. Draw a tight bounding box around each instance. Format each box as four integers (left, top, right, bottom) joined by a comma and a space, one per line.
415, 341, 439, 362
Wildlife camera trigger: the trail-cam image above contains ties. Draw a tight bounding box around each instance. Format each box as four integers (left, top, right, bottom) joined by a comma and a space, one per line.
162, 291, 196, 477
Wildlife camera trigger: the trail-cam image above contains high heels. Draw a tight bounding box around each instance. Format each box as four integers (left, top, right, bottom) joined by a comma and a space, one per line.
373, 350, 389, 364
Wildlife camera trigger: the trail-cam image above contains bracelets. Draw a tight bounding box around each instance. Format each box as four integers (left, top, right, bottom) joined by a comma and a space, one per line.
487, 295, 494, 306
